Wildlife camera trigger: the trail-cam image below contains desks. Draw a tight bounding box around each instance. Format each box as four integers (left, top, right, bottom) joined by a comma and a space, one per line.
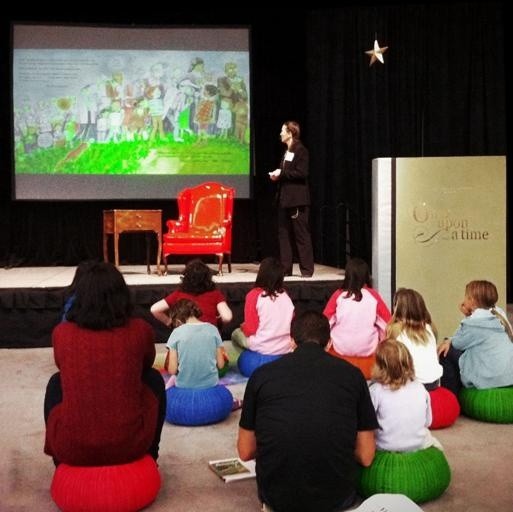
104, 209, 162, 275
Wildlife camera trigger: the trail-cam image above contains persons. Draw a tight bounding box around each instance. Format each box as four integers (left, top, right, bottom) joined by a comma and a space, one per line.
166, 298, 224, 388
232, 258, 296, 355
44, 263, 166, 466
148, 260, 232, 329
436, 280, 513, 392
365, 339, 441, 451
267, 121, 313, 279
237, 311, 380, 512
323, 258, 391, 355
385, 289, 442, 390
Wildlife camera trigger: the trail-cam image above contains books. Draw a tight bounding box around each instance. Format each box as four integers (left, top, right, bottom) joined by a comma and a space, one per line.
370, 155, 507, 350
210, 459, 256, 483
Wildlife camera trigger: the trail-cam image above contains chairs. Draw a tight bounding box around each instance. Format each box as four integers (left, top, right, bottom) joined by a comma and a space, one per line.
161, 182, 234, 276
